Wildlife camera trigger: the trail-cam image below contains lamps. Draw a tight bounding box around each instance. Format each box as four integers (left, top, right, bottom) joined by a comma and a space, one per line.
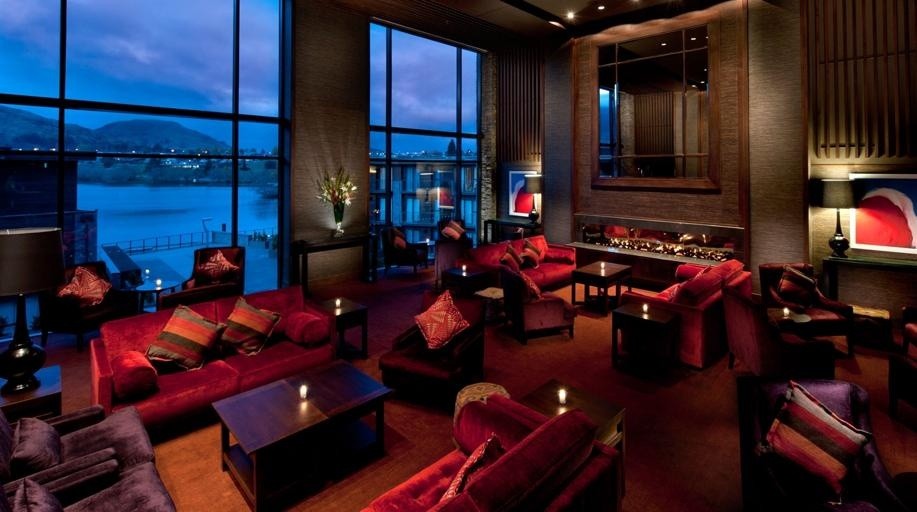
814, 178, 857, 256
523, 174, 544, 222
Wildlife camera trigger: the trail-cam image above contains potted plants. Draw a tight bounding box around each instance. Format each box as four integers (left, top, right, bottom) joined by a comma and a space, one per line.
314, 170, 356, 233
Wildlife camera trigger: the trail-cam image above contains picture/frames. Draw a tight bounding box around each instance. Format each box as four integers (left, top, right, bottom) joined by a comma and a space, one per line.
848, 173, 916, 254
508, 171, 536, 217
